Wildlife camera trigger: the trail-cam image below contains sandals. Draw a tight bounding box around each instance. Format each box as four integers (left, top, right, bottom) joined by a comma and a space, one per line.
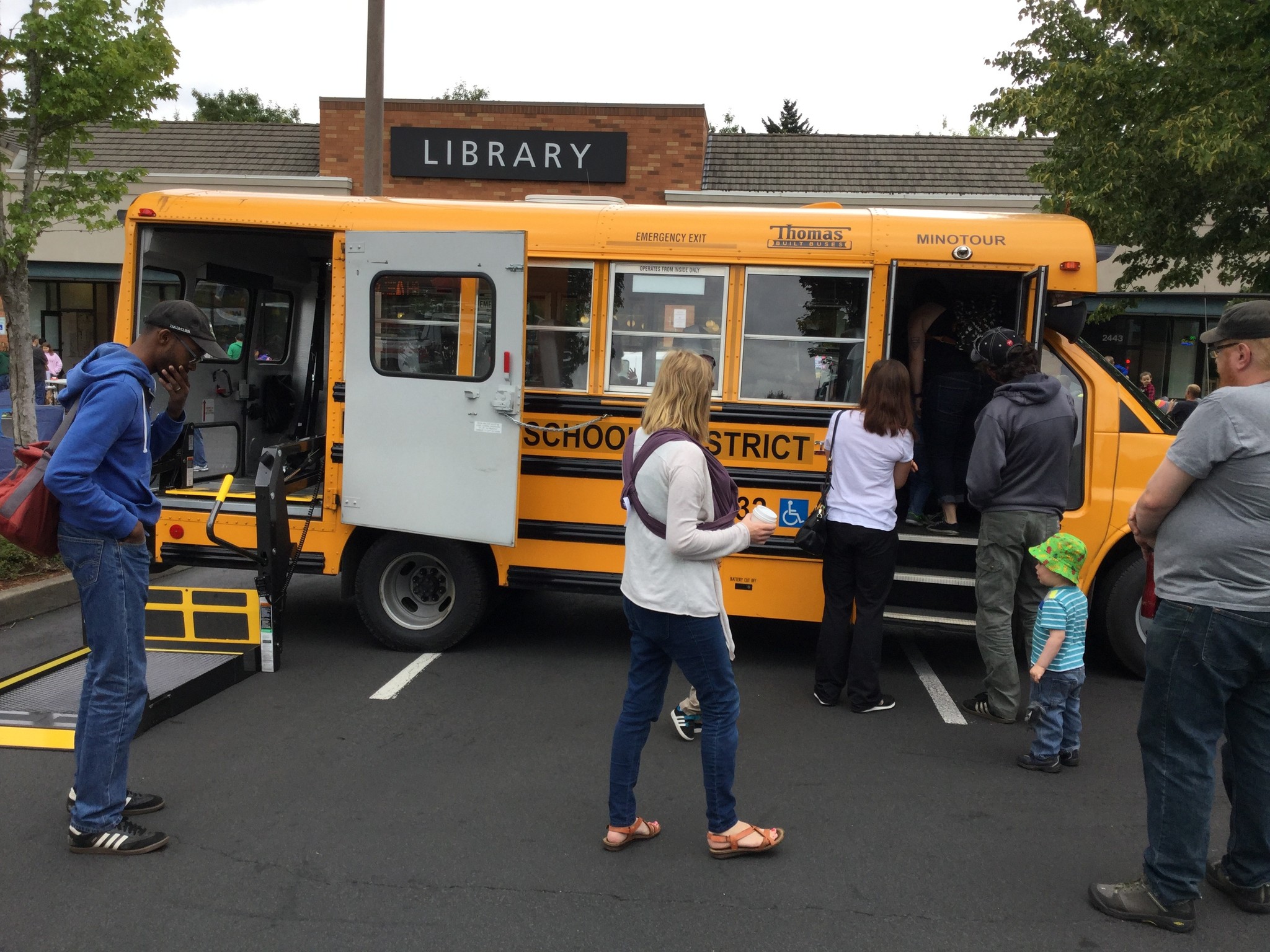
603, 817, 661, 851
706, 824, 785, 859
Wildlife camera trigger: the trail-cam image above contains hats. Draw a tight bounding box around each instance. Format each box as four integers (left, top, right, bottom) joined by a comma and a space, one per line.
143, 300, 229, 359
1200, 300, 1270, 343
1029, 533, 1087, 584
973, 326, 1023, 366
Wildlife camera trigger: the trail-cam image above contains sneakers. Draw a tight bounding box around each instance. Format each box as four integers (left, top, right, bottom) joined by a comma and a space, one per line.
66, 784, 165, 813
962, 699, 1014, 724
193, 464, 209, 472
1203, 856, 1270, 913
976, 692, 989, 701
68, 816, 169, 855
671, 704, 695, 741
694, 723, 703, 733
926, 518, 960, 535
905, 512, 924, 526
1059, 750, 1079, 766
814, 691, 831, 705
1088, 875, 1196, 933
862, 695, 895, 712
1017, 752, 1062, 773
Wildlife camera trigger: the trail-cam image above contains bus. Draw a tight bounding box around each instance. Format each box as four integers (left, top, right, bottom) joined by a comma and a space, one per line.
112, 201, 1185, 678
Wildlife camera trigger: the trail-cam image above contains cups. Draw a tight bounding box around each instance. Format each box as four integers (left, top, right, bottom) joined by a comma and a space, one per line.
751, 505, 778, 544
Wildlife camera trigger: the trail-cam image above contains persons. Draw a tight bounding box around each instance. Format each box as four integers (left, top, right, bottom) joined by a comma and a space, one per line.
0, 341, 10, 390
961, 327, 1078, 724
31, 333, 48, 406
1015, 532, 1091, 773
846, 341, 864, 404
814, 359, 919, 715
679, 319, 722, 383
227, 333, 244, 360
906, 277, 997, 535
41, 342, 63, 386
670, 685, 703, 741
1166, 384, 1201, 428
1105, 356, 1130, 379
565, 318, 638, 389
1139, 371, 1155, 404
602, 349, 785, 858
42, 300, 229, 856
1086, 300, 1269, 933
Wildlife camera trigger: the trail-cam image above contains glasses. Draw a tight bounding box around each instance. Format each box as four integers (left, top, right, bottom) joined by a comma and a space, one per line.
1208, 342, 1252, 359
700, 354, 716, 371
171, 332, 203, 365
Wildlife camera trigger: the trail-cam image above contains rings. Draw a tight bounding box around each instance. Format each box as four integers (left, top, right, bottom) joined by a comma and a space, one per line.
175, 387, 181, 392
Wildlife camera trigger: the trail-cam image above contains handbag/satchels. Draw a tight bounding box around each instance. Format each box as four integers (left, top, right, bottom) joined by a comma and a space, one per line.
0, 441, 60, 559
794, 505, 826, 558
59, 369, 65, 376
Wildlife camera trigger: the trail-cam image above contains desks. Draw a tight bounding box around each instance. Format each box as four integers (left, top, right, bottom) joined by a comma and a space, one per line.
45, 379, 67, 405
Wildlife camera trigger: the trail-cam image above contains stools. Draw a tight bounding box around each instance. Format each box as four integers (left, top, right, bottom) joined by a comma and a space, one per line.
45, 385, 56, 405
